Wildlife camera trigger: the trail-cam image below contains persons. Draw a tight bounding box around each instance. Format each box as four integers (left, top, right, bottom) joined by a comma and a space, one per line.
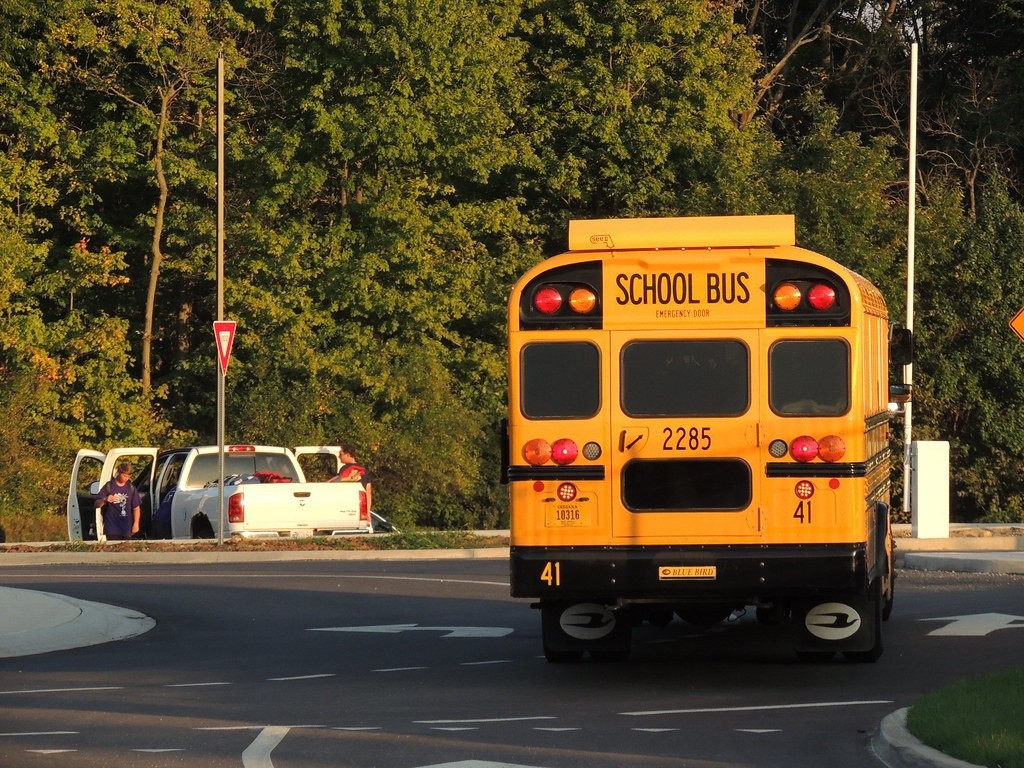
337, 444, 372, 522
93, 462, 140, 540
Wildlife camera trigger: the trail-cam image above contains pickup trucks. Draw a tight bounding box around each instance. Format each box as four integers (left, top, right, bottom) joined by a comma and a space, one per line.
66, 444, 375, 541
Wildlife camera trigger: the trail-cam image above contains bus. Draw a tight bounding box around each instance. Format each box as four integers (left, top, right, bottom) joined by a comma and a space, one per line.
496, 212, 916, 665
